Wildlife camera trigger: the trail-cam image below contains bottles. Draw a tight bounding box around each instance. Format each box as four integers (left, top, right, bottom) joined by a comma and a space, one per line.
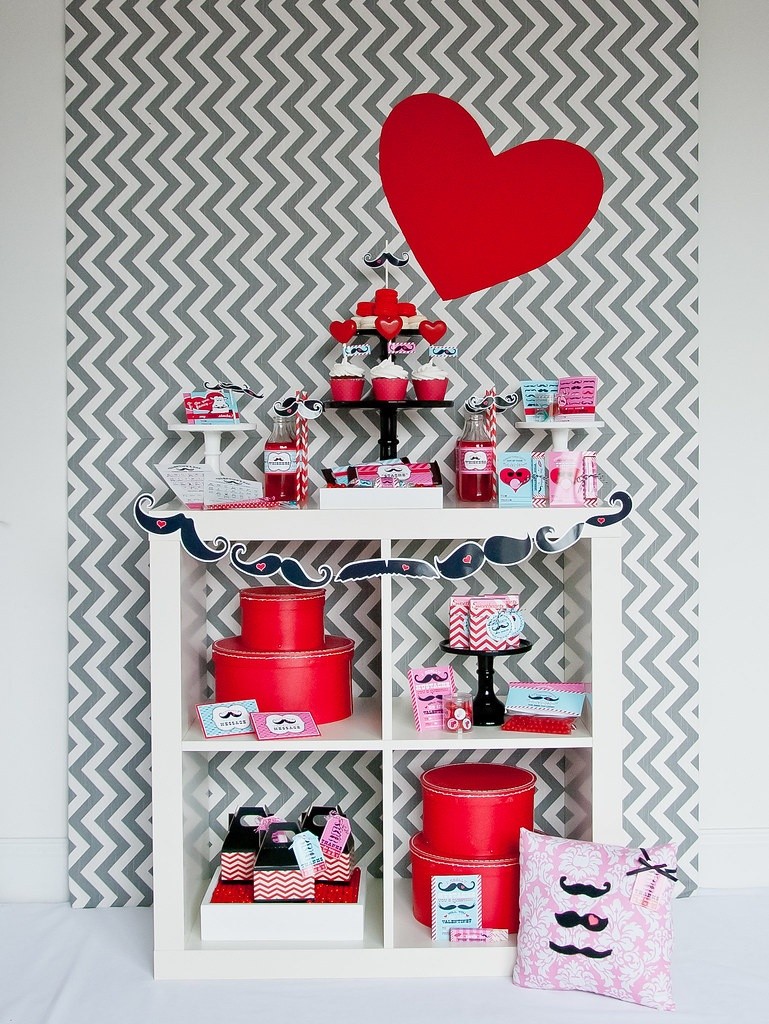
263, 416, 301, 503
456, 415, 493, 502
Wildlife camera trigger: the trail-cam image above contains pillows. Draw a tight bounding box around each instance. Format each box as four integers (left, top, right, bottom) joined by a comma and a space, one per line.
514, 824, 678, 1014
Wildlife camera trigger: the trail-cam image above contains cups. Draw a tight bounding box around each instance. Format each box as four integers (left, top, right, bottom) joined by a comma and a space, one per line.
442, 692, 473, 734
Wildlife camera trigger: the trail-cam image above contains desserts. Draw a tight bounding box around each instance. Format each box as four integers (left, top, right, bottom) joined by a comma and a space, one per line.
411, 363, 449, 400
370, 358, 409, 401
329, 361, 365, 401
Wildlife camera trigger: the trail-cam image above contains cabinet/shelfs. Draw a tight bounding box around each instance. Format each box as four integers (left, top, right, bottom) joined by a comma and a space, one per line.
148, 495, 620, 981
323, 328, 455, 461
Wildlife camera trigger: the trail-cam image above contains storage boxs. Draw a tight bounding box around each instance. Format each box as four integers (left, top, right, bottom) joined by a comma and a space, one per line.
201, 804, 366, 939
408, 829, 548, 934
213, 636, 355, 725
442, 593, 524, 734
420, 762, 537, 854
240, 585, 326, 651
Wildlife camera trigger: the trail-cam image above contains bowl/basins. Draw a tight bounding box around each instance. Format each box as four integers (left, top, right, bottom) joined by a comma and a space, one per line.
411, 378, 448, 400
372, 377, 409, 400
330, 379, 365, 401
